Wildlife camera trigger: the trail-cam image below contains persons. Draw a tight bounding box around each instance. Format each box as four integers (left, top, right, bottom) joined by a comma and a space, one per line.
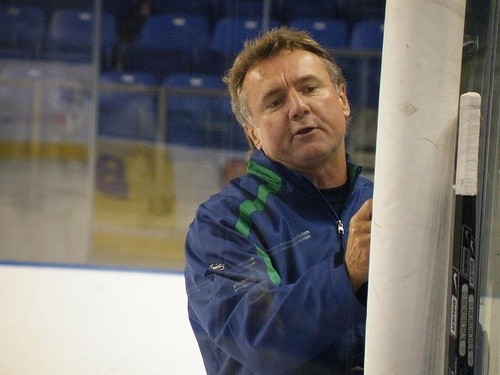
177, 28, 371, 375
